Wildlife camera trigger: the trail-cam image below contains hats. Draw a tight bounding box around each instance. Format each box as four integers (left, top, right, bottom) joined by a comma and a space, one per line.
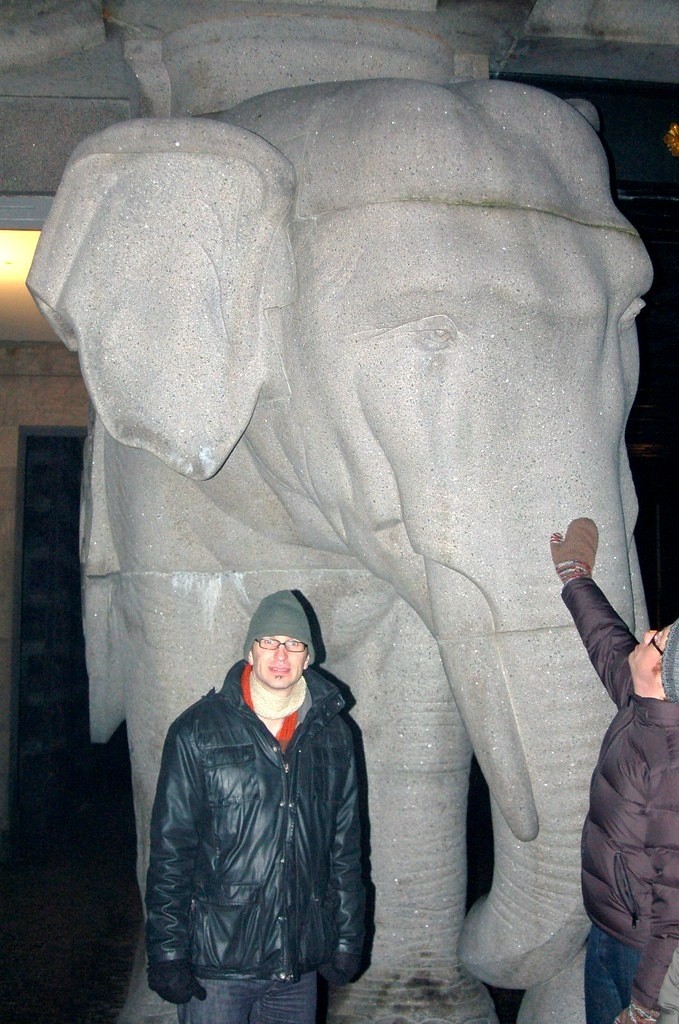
662, 617, 679, 703
243, 589, 315, 665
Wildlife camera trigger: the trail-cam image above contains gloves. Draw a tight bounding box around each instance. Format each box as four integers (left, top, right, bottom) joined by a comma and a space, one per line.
613, 995, 661, 1024
145, 964, 207, 1004
548, 517, 599, 582
318, 952, 358, 987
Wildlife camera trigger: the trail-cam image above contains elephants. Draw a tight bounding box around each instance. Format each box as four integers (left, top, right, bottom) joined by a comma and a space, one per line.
25, 79, 654, 1020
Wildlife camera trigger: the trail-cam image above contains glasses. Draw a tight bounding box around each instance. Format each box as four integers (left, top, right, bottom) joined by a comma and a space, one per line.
648, 630, 664, 656
255, 638, 308, 653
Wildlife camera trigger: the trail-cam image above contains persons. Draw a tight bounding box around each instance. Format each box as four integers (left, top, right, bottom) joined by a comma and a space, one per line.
145, 588, 368, 1024
548, 518, 678, 1024
